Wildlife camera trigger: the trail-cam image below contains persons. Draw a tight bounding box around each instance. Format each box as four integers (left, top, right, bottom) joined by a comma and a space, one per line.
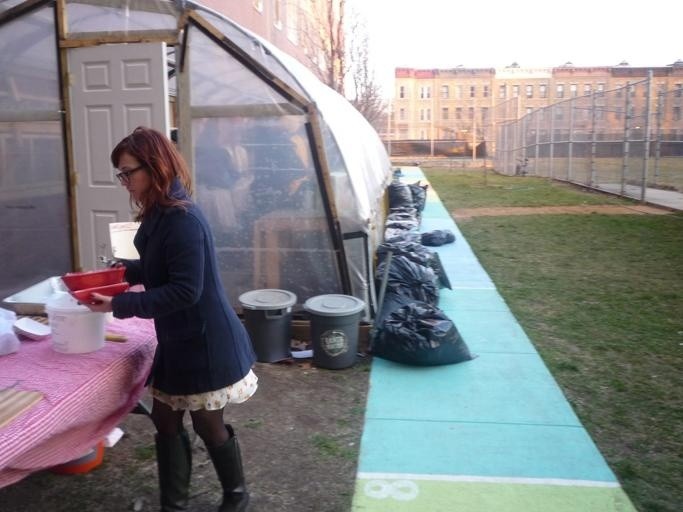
81, 126, 258, 512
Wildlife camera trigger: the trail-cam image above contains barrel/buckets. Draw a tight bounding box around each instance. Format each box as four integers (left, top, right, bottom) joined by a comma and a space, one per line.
45, 292, 106, 355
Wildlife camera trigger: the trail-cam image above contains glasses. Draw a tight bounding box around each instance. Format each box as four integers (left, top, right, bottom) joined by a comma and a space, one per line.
115, 164, 141, 184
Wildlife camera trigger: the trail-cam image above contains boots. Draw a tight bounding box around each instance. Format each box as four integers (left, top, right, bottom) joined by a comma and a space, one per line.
206, 425, 249, 512
154, 430, 193, 512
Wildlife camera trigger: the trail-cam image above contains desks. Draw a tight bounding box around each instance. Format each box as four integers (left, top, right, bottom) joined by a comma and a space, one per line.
0, 285, 159, 489
